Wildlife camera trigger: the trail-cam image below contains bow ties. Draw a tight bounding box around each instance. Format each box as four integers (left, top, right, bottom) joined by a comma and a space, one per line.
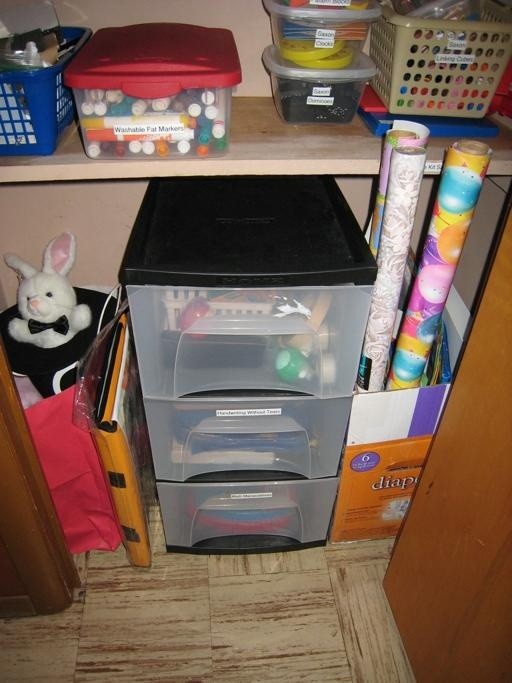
28, 314, 68, 336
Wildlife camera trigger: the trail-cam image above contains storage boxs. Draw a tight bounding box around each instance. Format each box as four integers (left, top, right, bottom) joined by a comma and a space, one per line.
260, 44, 379, 125
329, 282, 467, 549
64, 22, 243, 163
259, 0, 382, 69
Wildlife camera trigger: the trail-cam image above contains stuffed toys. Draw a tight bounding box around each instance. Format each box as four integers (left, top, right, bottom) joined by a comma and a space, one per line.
4, 231, 92, 347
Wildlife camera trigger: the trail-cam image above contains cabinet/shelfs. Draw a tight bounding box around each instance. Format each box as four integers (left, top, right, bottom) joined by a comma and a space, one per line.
119, 176, 375, 555
